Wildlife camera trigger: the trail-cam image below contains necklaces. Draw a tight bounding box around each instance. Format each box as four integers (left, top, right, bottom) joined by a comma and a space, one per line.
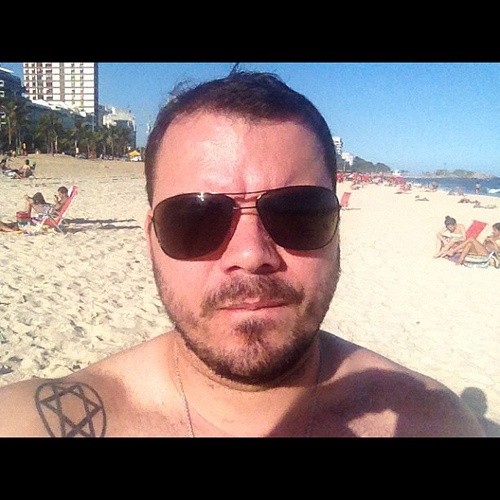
176, 340, 322, 438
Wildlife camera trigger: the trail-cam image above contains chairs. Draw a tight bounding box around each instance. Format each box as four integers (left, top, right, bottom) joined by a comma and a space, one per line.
24, 186, 77, 236
340, 192, 352, 210
449, 219, 500, 269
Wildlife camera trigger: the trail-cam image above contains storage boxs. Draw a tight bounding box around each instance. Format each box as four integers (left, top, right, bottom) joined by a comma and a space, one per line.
16, 211, 31, 223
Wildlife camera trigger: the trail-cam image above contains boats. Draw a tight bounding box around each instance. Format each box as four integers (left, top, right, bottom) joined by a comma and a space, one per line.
486, 187, 500, 194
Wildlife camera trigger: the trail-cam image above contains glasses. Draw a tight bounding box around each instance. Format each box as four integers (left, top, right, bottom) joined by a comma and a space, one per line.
147, 185, 341, 260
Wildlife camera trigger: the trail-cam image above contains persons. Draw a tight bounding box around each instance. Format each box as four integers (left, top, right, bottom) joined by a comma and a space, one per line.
448, 222, 500, 264
25, 187, 70, 218
339, 173, 411, 192
2, 71, 486, 437
0, 152, 11, 168
432, 214, 466, 258
10, 159, 31, 178
476, 182, 480, 193
